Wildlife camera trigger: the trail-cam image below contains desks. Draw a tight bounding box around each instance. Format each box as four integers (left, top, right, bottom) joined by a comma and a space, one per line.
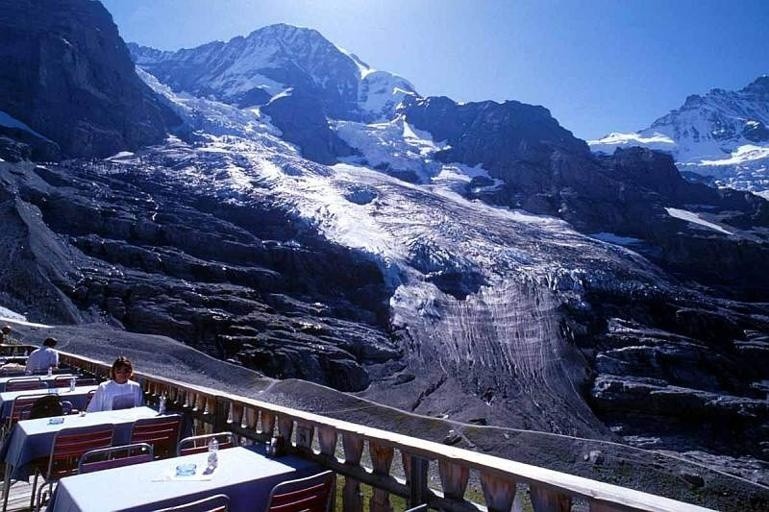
3, 405, 169, 512
46, 443, 318, 512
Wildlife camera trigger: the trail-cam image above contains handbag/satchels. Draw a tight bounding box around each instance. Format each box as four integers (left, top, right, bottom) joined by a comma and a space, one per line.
31, 396, 64, 418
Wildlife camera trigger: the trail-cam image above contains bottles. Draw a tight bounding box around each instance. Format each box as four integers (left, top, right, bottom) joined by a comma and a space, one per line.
207, 437, 218, 471
70, 375, 76, 391
159, 393, 167, 416
48, 365, 53, 378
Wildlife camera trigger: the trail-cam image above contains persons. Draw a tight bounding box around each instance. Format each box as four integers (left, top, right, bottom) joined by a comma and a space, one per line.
0, 325, 12, 343
86, 356, 145, 412
26, 337, 60, 371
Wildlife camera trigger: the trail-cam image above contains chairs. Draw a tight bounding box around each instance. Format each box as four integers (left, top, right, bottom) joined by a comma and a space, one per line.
28, 421, 117, 512
264, 468, 335, 512
0, 391, 62, 458
154, 493, 231, 512
127, 413, 184, 460
77, 442, 155, 475
2, 353, 100, 415
176, 430, 239, 457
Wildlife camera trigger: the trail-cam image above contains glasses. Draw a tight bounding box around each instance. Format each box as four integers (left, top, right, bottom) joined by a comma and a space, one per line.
116, 368, 131, 374
